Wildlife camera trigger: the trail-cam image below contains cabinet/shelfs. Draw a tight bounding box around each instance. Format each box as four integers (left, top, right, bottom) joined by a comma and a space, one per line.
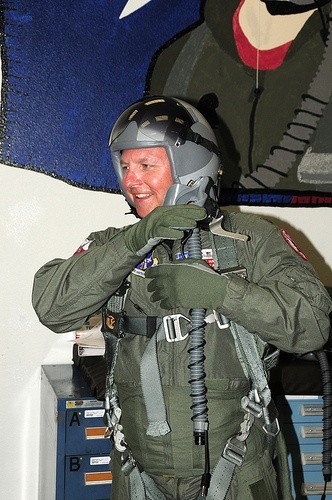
40, 364, 332, 500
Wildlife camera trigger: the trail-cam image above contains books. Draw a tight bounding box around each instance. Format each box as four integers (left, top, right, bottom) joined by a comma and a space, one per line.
75, 310, 107, 358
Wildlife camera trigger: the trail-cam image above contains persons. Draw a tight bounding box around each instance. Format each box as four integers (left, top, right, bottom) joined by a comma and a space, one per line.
31, 92, 332, 500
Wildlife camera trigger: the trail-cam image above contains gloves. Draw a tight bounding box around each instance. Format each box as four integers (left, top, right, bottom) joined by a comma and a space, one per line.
124, 204, 207, 257
144, 259, 230, 309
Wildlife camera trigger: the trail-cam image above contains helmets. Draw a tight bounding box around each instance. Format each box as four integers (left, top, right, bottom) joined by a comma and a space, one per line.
108, 95, 222, 220
